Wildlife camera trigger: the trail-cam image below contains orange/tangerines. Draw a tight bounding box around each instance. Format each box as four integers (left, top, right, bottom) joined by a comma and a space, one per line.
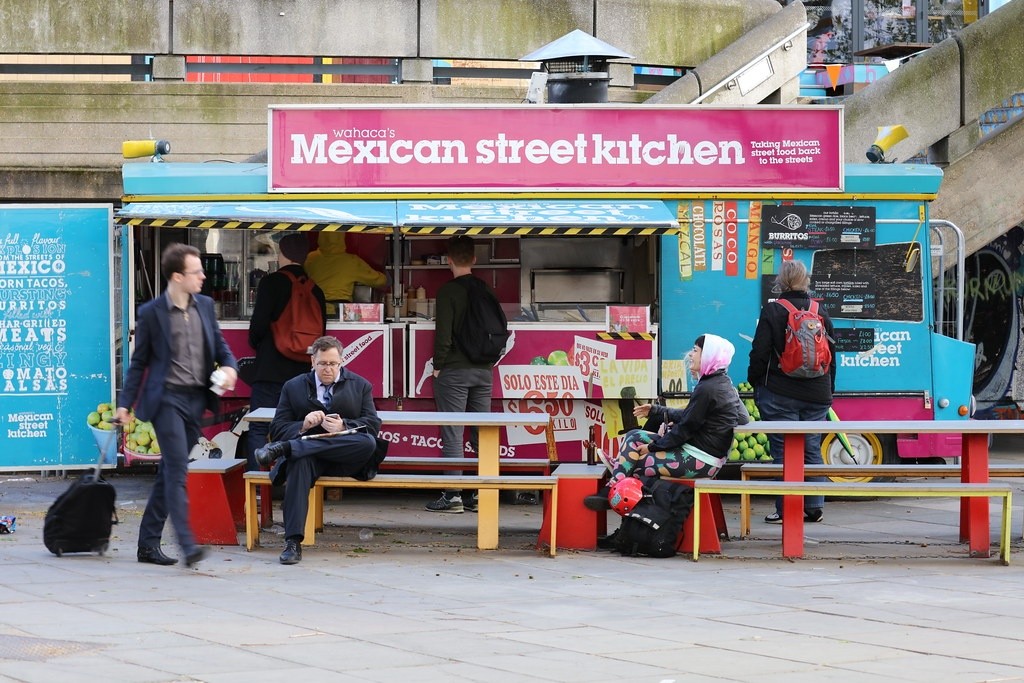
86, 403, 161, 456
726, 381, 773, 460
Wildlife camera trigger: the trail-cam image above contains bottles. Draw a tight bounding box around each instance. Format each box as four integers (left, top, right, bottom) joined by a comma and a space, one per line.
397, 397, 402, 411
587, 426, 597, 465
408, 285, 426, 299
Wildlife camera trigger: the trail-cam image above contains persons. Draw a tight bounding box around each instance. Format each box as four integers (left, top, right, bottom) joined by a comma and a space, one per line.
112, 244, 239, 568
424, 234, 494, 514
747, 259, 837, 524
583, 333, 740, 547
305, 230, 387, 319
254, 337, 382, 565
247, 233, 327, 499
633, 357, 749, 433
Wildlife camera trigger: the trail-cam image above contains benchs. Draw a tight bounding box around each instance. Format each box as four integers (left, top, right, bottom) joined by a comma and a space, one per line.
740, 463, 1024, 539
243, 470, 559, 556
694, 479, 1013, 565
261, 457, 551, 529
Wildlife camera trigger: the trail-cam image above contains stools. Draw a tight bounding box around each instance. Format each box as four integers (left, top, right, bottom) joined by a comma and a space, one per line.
659, 474, 730, 554
185, 458, 247, 545
535, 463, 607, 551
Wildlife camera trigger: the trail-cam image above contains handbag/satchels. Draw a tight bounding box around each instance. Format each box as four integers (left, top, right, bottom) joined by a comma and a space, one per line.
352, 439, 389, 481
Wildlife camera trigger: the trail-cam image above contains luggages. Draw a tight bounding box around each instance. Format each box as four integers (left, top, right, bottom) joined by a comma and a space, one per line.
45, 416, 121, 555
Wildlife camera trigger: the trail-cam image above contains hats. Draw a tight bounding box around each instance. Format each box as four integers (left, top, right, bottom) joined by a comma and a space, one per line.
280, 234, 309, 263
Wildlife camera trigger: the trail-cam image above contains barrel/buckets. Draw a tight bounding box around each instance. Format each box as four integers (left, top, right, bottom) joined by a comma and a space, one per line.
87, 418, 116, 463
387, 240, 411, 265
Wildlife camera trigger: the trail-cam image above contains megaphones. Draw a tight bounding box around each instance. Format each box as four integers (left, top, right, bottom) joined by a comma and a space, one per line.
866, 125, 909, 162
122, 140, 171, 159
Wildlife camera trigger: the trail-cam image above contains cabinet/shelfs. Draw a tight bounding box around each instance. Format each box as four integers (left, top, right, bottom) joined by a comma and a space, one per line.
186, 228, 281, 317
381, 233, 524, 321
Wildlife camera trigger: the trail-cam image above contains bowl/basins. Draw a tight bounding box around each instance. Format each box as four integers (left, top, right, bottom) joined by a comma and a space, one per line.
411, 260, 424, 265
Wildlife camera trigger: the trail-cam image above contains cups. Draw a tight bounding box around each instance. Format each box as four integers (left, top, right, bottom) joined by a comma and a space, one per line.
249, 290, 253, 302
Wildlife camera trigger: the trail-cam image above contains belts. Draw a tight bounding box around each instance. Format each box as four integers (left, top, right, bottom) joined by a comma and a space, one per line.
164, 382, 208, 393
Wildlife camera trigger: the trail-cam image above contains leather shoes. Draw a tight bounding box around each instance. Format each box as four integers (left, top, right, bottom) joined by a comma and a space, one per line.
137, 546, 179, 565
279, 538, 303, 564
185, 545, 212, 565
255, 441, 285, 468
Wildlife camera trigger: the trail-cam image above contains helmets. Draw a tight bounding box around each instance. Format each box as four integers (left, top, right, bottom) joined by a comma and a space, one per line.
608, 476, 642, 517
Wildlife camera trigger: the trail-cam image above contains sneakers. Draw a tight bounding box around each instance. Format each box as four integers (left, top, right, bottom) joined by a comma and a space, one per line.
803, 508, 824, 522
425, 490, 464, 513
597, 528, 621, 551
463, 493, 478, 512
765, 511, 783, 524
584, 485, 614, 510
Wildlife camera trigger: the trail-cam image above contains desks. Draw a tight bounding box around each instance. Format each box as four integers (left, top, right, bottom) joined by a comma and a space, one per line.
734, 420, 1024, 557
243, 407, 549, 549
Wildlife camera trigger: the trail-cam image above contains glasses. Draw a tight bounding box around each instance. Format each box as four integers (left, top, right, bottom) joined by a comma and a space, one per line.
311, 357, 341, 370
176, 268, 204, 280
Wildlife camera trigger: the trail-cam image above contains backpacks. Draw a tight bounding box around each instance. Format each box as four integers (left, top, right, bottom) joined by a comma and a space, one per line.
613, 479, 694, 559
445, 279, 508, 365
771, 297, 835, 378
270, 270, 324, 362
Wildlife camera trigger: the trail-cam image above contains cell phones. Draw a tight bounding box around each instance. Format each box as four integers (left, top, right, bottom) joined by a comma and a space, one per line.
326, 414, 338, 422
